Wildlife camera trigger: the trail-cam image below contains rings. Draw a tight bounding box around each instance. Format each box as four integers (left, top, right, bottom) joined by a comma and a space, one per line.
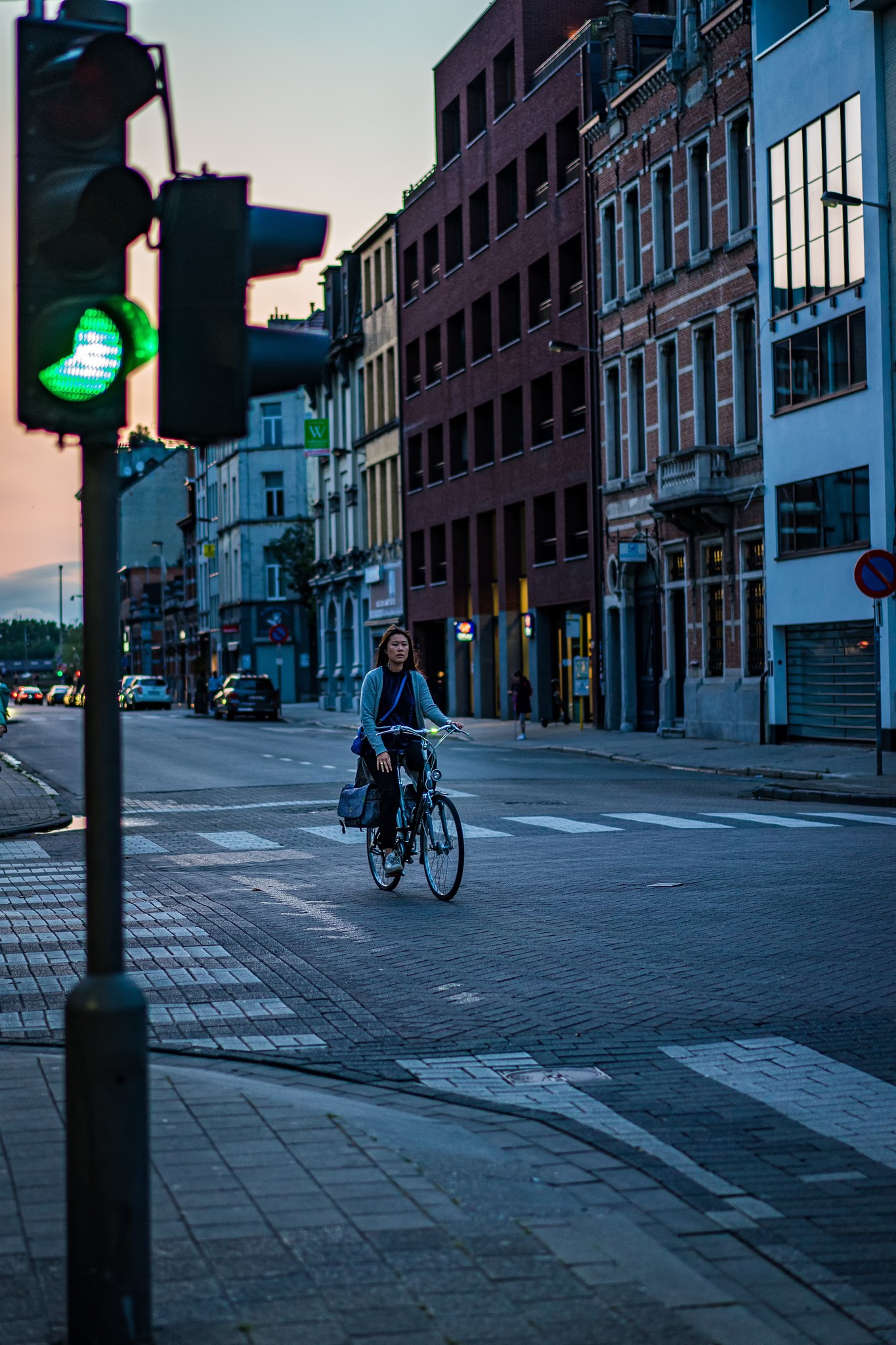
380, 762, 383, 764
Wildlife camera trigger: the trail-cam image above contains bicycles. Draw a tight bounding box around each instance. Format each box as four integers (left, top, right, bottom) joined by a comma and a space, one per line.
366, 720, 470, 902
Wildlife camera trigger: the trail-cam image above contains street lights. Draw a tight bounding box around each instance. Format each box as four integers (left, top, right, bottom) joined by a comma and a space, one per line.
152, 540, 167, 681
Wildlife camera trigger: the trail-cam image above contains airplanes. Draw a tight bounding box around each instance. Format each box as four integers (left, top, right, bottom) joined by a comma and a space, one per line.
12, 12, 161, 439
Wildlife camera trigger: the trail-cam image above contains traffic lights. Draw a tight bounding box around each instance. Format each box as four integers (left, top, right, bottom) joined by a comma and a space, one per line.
158, 175, 335, 448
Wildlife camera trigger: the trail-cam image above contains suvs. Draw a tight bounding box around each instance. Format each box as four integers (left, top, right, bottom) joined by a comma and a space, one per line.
118, 674, 172, 710
213, 673, 279, 722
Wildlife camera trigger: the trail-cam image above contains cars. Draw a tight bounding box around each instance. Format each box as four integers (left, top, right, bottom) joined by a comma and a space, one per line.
13, 683, 85, 705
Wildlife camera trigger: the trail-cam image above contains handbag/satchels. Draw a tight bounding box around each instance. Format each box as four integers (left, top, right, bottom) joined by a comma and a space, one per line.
351, 725, 367, 756
337, 784, 380, 828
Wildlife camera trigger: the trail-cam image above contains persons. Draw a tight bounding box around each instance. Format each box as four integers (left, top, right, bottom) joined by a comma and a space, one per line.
0, 683, 11, 738
208, 669, 224, 712
359, 625, 465, 875
506, 670, 533, 741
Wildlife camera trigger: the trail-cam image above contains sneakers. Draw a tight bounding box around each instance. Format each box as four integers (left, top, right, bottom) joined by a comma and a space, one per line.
384, 852, 404, 874
411, 807, 421, 835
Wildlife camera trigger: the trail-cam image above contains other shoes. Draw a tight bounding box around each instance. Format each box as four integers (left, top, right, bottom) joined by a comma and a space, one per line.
517, 733, 527, 740
